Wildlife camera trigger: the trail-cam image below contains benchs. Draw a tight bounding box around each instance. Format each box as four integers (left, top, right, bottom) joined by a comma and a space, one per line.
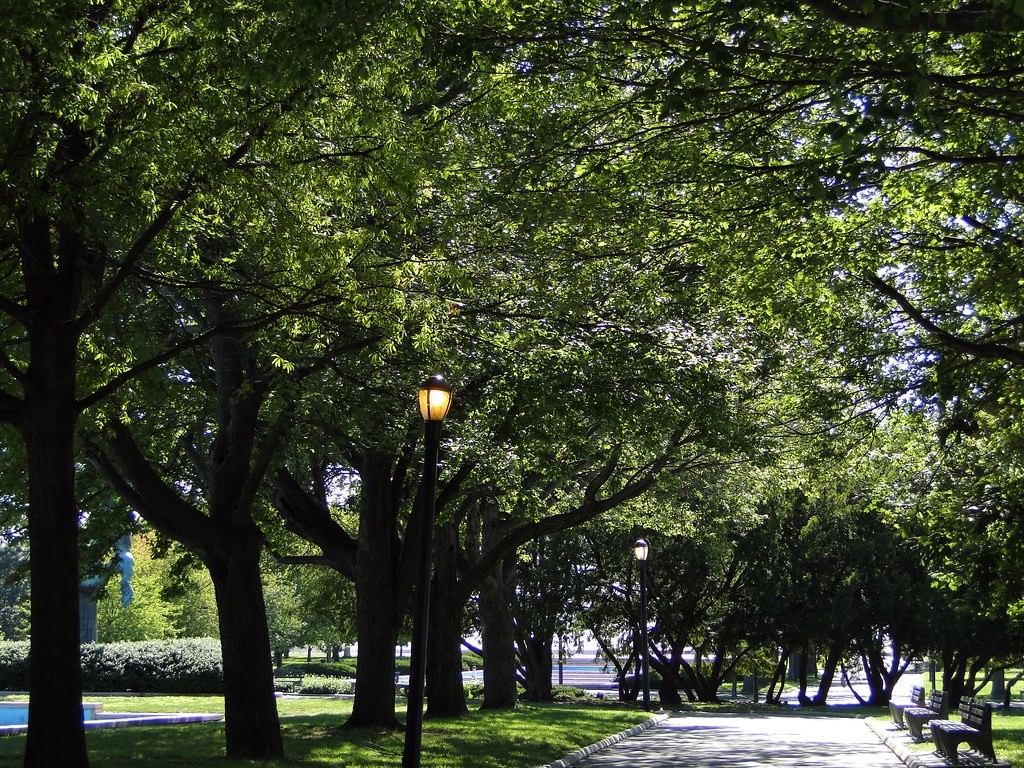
928, 696, 999, 764
349, 672, 401, 694
889, 686, 925, 729
275, 669, 305, 688
904, 689, 948, 740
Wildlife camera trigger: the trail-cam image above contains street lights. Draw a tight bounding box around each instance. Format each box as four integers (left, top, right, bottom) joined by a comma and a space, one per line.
401, 371, 456, 768
634, 539, 652, 713
